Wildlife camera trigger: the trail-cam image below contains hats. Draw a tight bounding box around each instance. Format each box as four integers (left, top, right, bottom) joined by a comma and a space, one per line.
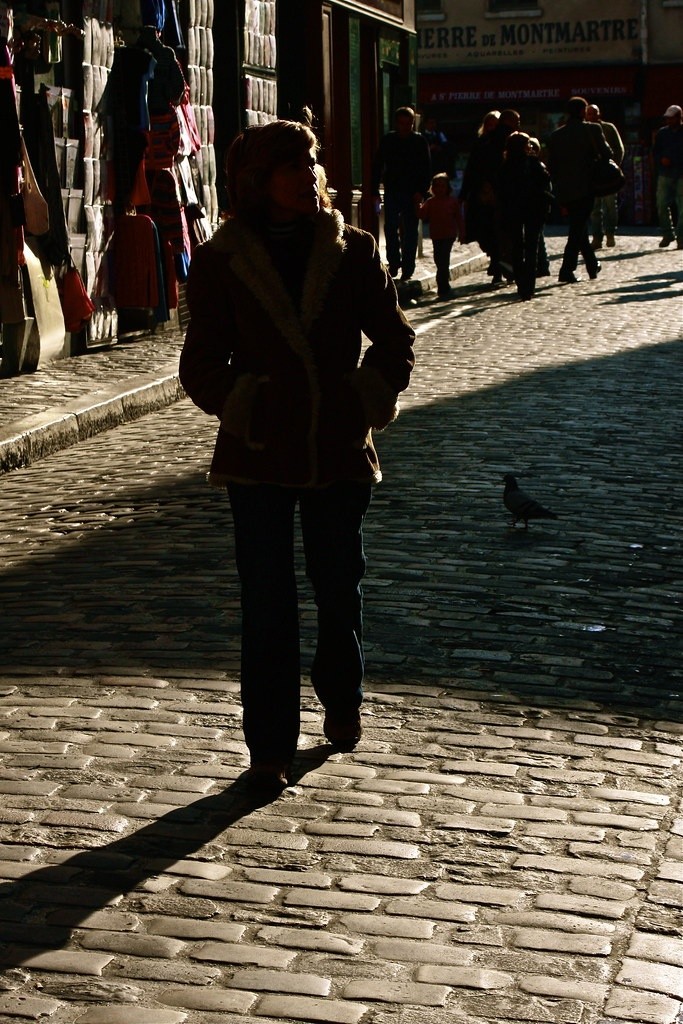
663, 105, 682, 117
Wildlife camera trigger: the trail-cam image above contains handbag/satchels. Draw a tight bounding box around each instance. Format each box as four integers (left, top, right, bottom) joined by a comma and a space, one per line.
586, 121, 625, 197
20, 136, 49, 236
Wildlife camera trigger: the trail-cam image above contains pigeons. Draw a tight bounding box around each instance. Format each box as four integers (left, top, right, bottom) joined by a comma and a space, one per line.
500, 474, 559, 528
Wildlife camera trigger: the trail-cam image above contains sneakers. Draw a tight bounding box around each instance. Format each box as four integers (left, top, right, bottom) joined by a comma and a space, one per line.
248, 758, 287, 787
324, 707, 361, 746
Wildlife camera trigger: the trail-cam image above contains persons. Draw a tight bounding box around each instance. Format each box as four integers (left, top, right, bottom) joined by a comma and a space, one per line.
652, 105, 683, 248
178, 121, 416, 786
585, 104, 625, 248
458, 110, 552, 302
371, 107, 431, 280
422, 116, 447, 174
546, 96, 614, 283
415, 172, 465, 296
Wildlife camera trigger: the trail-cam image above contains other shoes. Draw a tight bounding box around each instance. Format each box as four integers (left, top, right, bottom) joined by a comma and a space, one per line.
402, 268, 413, 279
607, 234, 614, 246
437, 283, 450, 297
487, 258, 549, 301
677, 239, 683, 249
559, 273, 577, 282
659, 233, 674, 247
388, 257, 400, 277
590, 238, 602, 249
588, 266, 601, 279
